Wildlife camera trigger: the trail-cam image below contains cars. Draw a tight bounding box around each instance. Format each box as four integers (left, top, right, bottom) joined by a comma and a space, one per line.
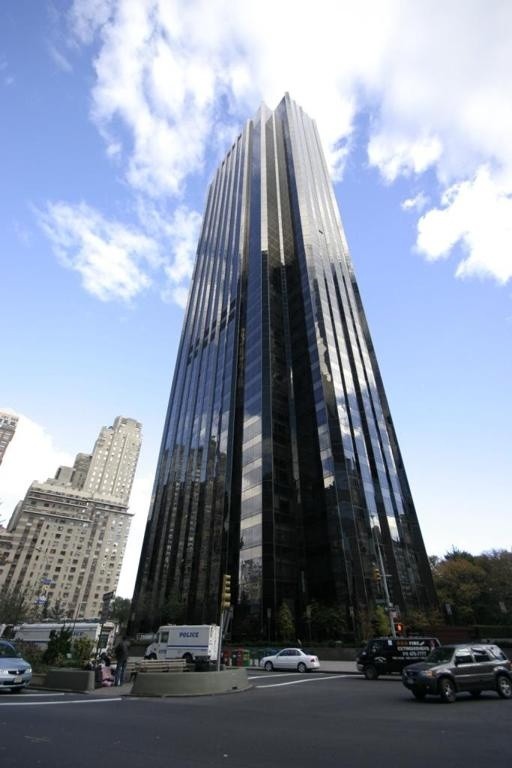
260, 648, 320, 673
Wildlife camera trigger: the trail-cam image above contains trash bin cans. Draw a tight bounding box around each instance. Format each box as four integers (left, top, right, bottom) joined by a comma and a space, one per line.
195, 656, 211, 671
231, 649, 250, 666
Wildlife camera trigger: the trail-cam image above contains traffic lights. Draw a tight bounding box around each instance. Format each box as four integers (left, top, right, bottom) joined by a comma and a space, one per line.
395, 624, 403, 632
222, 574, 232, 609
374, 568, 381, 582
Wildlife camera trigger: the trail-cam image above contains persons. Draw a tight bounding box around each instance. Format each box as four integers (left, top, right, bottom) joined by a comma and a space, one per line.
114, 636, 131, 686
91, 650, 115, 685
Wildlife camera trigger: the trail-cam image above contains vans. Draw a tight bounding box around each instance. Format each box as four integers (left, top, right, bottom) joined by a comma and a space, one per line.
356, 636, 441, 680
0, 639, 32, 692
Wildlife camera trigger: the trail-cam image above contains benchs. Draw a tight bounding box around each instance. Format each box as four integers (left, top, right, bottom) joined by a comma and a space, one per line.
129, 659, 186, 682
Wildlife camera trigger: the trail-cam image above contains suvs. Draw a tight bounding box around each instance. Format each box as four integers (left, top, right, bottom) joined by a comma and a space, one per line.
403, 644, 512, 702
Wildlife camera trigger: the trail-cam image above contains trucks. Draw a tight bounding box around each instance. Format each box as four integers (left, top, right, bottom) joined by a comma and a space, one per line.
1, 622, 116, 658
144, 624, 223, 663
